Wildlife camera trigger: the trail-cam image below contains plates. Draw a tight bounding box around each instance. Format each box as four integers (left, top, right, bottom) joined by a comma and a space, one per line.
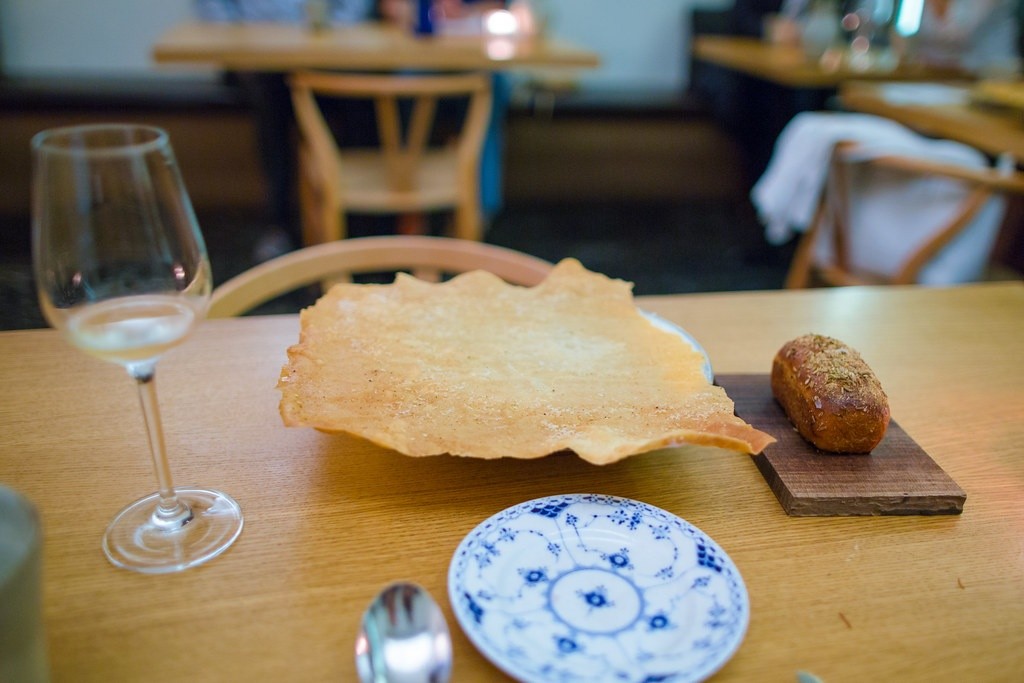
636, 307, 713, 387
448, 493, 750, 683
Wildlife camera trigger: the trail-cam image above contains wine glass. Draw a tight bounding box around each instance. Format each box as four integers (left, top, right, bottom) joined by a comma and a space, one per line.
31, 123, 245, 574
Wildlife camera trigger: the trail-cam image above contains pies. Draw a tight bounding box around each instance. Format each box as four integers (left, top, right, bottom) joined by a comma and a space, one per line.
278, 256, 781, 466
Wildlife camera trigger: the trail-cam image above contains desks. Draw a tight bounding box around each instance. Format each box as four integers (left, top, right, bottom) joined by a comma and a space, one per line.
694, 35, 978, 87
156, 22, 598, 67
841, 80, 1024, 166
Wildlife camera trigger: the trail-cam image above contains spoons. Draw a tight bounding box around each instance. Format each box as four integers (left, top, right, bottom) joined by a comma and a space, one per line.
355, 580, 453, 683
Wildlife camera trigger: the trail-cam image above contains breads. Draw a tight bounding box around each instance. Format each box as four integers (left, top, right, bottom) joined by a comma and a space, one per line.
771, 334, 890, 455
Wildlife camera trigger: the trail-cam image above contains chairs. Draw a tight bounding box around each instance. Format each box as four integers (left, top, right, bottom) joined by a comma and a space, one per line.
286, 69, 489, 298
780, 140, 1024, 289
202, 235, 558, 319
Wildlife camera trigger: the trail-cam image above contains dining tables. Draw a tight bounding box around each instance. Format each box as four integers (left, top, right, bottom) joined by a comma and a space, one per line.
0, 283, 1023, 683
